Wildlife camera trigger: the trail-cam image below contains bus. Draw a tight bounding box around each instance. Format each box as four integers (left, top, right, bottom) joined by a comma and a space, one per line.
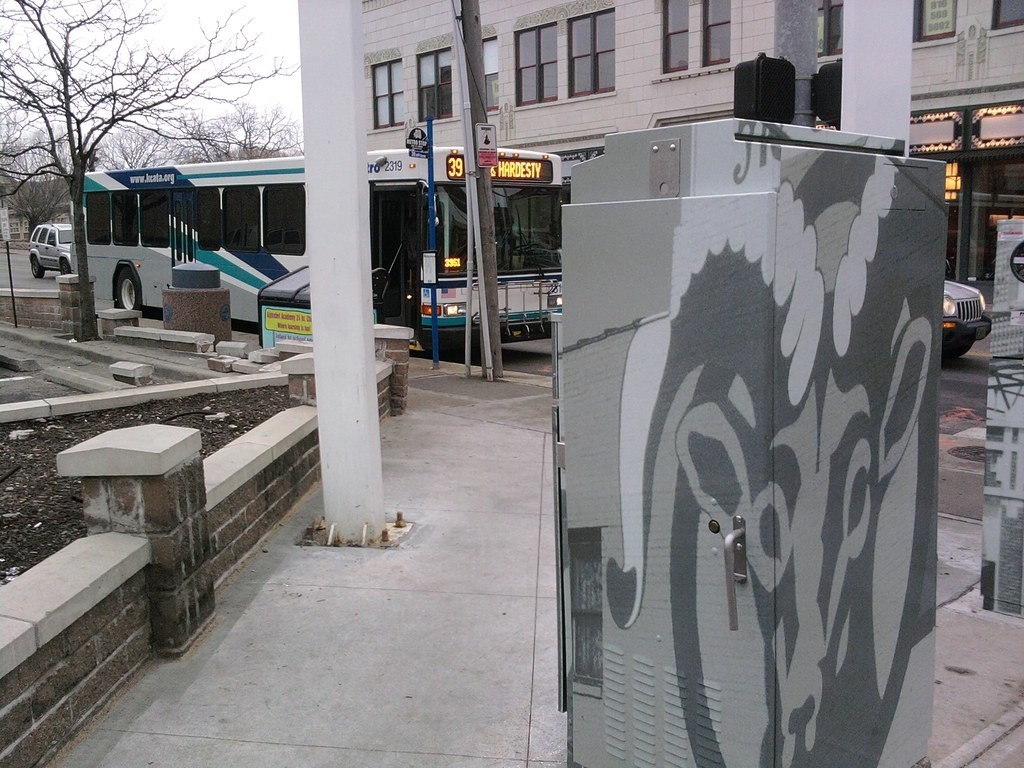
71, 147, 564, 350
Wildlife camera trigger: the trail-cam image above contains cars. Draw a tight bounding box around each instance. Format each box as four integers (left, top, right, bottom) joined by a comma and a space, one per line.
507, 244, 546, 255
230, 226, 303, 252
943, 280, 992, 359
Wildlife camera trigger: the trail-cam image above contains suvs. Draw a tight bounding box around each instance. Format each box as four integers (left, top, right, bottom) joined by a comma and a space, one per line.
27, 222, 73, 278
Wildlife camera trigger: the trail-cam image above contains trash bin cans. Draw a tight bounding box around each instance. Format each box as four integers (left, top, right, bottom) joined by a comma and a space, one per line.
257, 265, 387, 354
